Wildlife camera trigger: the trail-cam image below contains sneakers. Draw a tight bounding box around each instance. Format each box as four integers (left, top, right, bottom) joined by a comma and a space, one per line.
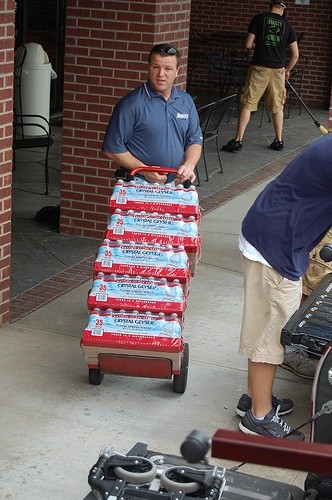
238, 405, 305, 441
278, 344, 315, 380
235, 392, 295, 418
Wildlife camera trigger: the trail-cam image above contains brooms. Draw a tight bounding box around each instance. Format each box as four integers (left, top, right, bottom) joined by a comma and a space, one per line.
286, 81, 328, 135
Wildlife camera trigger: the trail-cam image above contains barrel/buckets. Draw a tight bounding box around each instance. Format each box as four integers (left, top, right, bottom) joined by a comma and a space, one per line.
19, 44, 58, 136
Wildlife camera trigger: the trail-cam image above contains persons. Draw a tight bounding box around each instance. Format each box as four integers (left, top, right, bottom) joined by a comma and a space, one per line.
221, 0, 300, 151
237, 131, 331, 443
99, 42, 204, 190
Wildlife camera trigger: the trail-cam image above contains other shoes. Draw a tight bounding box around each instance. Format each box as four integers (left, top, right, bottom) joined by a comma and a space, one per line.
270, 138, 283, 151
222, 139, 243, 151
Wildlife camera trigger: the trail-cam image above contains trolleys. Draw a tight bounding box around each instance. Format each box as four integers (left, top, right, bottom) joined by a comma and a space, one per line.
89, 166, 189, 394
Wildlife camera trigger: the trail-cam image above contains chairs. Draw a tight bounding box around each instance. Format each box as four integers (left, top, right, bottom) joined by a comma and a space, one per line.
12, 42, 54, 195
186, 28, 308, 186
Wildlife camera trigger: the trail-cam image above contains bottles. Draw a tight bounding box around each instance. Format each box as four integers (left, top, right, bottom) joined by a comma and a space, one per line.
84, 308, 181, 349
108, 178, 200, 227
94, 238, 189, 279
88, 271, 184, 312
103, 208, 199, 252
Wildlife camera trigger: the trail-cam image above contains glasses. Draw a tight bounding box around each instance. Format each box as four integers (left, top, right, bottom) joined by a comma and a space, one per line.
151, 45, 178, 55
